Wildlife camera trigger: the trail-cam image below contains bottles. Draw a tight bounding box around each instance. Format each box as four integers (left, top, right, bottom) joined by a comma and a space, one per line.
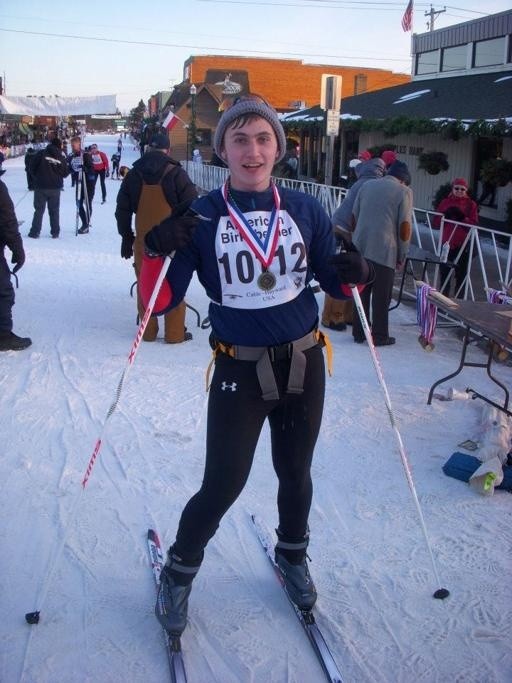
440, 241, 450, 263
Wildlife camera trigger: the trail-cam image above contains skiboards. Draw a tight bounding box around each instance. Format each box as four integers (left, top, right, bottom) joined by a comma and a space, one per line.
148, 515, 343, 683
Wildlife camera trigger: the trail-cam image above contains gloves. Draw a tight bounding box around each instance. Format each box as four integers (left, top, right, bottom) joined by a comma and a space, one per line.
147, 216, 197, 257
444, 205, 465, 222
106, 171, 109, 177
337, 250, 370, 284
120, 225, 137, 259
9, 238, 25, 273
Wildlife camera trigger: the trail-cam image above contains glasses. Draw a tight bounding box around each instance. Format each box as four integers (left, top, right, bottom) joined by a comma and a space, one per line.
454, 186, 468, 191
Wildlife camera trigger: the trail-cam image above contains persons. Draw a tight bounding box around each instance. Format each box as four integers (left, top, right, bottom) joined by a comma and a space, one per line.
321, 148, 414, 347
431, 177, 479, 299
24, 131, 128, 242
281, 146, 298, 179
208, 147, 228, 167
0, 153, 33, 353
137, 94, 376, 625
114, 133, 197, 343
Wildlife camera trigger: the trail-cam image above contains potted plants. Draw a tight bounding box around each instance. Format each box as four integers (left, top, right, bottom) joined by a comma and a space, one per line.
479, 157, 512, 186
419, 152, 450, 175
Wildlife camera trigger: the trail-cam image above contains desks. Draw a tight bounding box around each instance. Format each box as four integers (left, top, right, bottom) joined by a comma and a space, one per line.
389, 246, 458, 311
416, 299, 512, 416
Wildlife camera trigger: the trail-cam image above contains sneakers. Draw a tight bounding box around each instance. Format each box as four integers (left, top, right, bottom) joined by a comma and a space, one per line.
375, 337, 396, 347
323, 318, 349, 331
184, 332, 192, 339
1, 332, 32, 351
79, 226, 90, 234
29, 234, 59, 238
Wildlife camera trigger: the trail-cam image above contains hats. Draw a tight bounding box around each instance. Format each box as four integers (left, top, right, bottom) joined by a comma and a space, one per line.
387, 160, 412, 185
381, 150, 397, 165
214, 93, 287, 166
358, 151, 373, 160
452, 178, 468, 186
149, 134, 171, 150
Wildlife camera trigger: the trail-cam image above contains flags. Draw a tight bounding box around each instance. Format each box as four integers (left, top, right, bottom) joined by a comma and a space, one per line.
162, 110, 177, 130
402, 1, 413, 31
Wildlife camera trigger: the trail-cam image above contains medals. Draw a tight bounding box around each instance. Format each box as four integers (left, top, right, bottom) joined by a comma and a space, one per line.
257, 272, 276, 291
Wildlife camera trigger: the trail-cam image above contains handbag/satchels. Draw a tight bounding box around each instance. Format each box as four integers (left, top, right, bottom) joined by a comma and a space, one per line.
461, 237, 481, 261
23, 148, 52, 192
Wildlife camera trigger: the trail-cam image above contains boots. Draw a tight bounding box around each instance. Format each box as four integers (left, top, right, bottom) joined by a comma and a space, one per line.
274, 523, 319, 610
154, 543, 202, 634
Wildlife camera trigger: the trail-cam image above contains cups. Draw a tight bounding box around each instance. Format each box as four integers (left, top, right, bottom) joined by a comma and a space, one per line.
448, 388, 469, 400
